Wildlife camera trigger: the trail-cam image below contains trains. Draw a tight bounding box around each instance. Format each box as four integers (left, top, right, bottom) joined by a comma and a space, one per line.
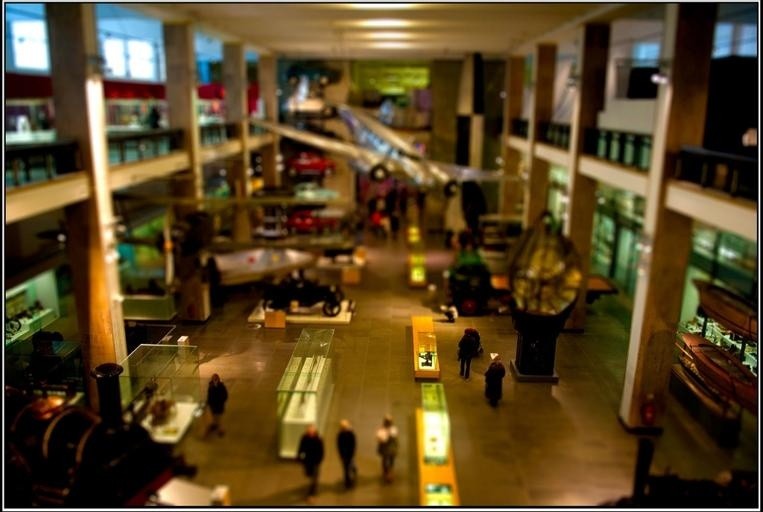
3, 327, 236, 508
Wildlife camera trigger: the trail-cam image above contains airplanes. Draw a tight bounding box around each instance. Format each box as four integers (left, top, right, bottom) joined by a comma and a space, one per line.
236, 92, 525, 196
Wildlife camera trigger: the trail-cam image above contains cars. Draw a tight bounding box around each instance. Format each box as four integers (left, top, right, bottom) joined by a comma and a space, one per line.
472, 211, 525, 275
260, 271, 347, 319
254, 58, 342, 239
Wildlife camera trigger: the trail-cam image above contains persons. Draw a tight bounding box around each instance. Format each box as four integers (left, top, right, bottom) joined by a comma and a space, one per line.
361, 181, 426, 241
482, 356, 505, 408
206, 373, 229, 437
457, 328, 481, 380
296, 422, 325, 504
201, 257, 223, 316
374, 413, 400, 484
146, 105, 161, 129
735, 127, 758, 197
336, 419, 358, 492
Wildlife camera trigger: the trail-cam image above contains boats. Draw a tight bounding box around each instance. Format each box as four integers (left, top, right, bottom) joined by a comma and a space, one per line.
667, 274, 759, 442
509, 207, 586, 340
